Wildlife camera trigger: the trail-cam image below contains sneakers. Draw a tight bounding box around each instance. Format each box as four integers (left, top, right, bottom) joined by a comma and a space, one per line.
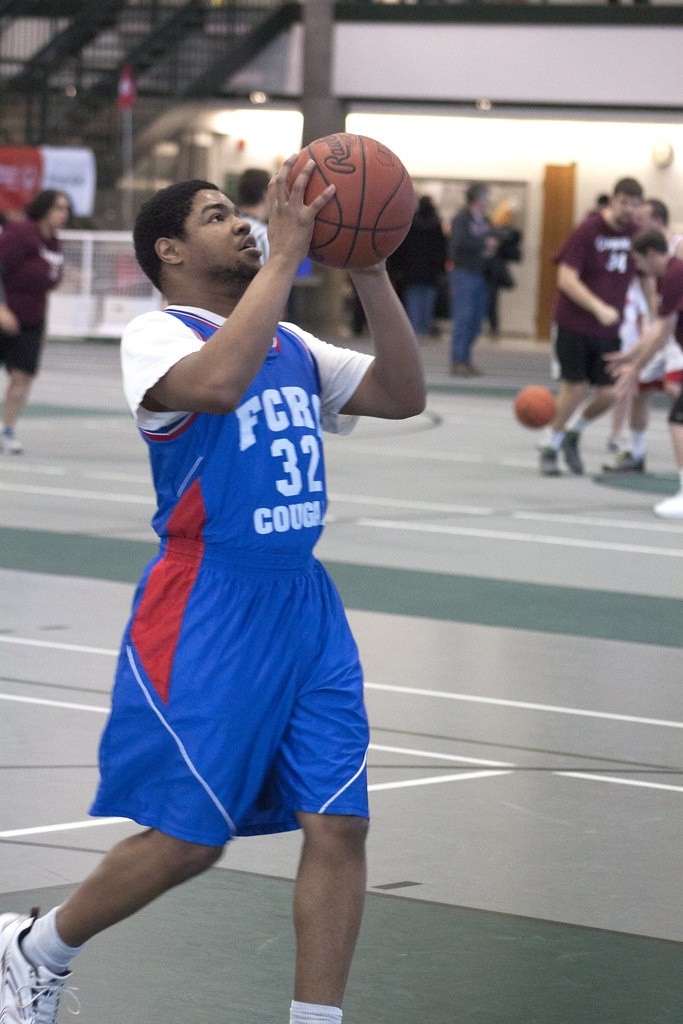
0, 907, 80, 1024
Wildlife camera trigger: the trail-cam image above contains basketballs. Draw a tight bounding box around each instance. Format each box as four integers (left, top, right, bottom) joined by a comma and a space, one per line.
274, 131, 416, 270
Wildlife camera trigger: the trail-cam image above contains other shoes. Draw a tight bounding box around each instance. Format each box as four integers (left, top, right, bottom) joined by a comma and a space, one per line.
0, 427, 22, 451
562, 432, 583, 474
450, 363, 479, 379
654, 491, 683, 519
602, 450, 645, 471
602, 440, 620, 454
534, 442, 561, 475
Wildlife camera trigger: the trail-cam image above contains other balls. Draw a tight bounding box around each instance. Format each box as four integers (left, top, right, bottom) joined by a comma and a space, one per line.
514, 386, 557, 427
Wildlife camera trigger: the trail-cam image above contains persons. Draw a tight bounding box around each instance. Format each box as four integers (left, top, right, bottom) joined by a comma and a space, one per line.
234, 168, 682, 521
1, 180, 426, 1023
1, 189, 74, 452
